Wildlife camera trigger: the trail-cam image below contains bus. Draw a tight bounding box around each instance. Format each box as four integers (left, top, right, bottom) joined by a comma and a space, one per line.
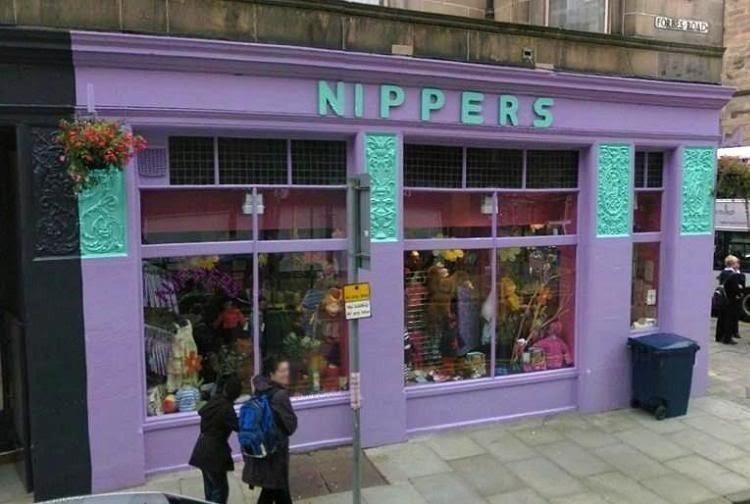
712, 199, 750, 322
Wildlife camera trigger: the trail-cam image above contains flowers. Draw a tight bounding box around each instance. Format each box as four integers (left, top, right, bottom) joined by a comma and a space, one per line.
49, 113, 148, 195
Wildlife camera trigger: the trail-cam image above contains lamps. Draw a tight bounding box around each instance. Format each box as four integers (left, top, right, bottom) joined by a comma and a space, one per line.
481, 196, 499, 216
241, 192, 266, 216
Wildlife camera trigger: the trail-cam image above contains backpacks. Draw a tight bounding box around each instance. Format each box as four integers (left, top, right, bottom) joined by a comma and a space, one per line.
711, 272, 737, 318
238, 386, 285, 461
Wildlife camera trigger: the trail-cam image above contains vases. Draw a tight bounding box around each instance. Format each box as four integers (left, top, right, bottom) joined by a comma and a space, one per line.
85, 160, 109, 170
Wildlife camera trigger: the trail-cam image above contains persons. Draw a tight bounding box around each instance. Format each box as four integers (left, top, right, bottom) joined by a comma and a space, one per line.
716, 254, 740, 345
242, 353, 297, 504
732, 264, 746, 338
189, 377, 243, 504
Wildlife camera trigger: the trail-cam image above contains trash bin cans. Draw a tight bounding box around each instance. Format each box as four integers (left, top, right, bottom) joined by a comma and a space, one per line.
627, 333, 700, 420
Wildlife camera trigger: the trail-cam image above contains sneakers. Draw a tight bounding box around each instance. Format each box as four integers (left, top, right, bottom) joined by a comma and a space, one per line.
715, 331, 741, 344
